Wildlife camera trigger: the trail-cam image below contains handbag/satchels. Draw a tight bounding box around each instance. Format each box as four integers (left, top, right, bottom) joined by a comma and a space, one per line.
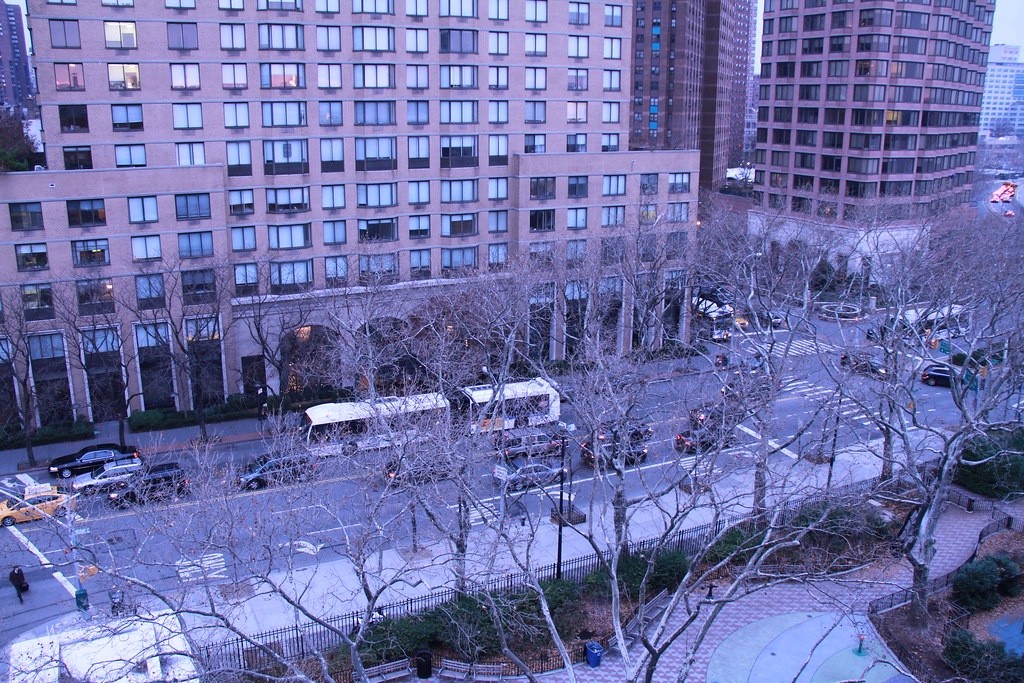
21, 581, 29, 593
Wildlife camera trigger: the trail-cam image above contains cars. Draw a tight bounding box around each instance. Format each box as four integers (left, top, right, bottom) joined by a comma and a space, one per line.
673, 424, 735, 455
921, 363, 976, 390
235, 448, 310, 492
580, 418, 654, 471
688, 359, 782, 430
47, 443, 139, 480
495, 427, 569, 461
109, 463, 188, 512
491, 459, 568, 493
382, 448, 466, 486
727, 302, 749, 330
743, 305, 781, 328
71, 457, 148, 496
839, 353, 893, 380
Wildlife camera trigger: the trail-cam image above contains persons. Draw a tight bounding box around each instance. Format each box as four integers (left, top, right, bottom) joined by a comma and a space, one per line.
9, 566, 25, 604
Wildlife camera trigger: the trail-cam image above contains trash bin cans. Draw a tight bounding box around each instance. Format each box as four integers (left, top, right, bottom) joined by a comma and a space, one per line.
586, 642, 604, 668
416, 651, 433, 678
75, 588, 89, 611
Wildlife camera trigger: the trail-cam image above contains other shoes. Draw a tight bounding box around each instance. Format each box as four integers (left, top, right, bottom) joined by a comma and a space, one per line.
20, 600, 23, 605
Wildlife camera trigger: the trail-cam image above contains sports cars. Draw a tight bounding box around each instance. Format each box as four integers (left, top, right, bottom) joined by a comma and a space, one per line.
0, 485, 77, 528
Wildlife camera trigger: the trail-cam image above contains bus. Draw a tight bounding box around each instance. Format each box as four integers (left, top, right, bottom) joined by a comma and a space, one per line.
297, 392, 452, 460
885, 303, 973, 348
692, 296, 733, 343
455, 377, 562, 434
